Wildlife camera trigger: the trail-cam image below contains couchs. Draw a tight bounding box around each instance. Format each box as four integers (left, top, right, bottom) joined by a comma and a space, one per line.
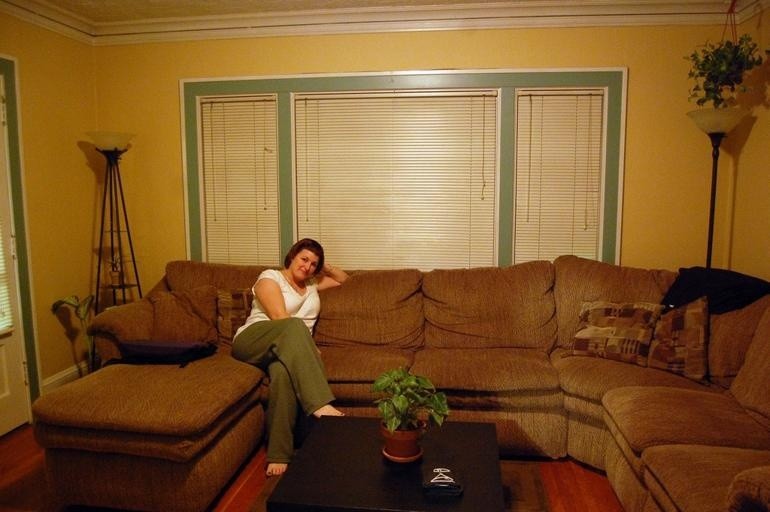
410, 260, 568, 461
30, 253, 424, 511
551, 254, 770, 471
601, 308, 770, 511
631, 444, 770, 511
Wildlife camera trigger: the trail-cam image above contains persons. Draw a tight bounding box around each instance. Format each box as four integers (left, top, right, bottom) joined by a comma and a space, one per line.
231, 238, 351, 476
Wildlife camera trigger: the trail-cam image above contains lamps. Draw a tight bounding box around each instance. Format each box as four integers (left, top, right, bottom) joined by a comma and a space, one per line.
686, 108, 751, 270
83, 129, 142, 317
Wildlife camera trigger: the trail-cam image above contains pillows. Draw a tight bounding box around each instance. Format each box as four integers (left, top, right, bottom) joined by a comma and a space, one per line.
648, 296, 713, 387
217, 286, 257, 345
560, 300, 662, 368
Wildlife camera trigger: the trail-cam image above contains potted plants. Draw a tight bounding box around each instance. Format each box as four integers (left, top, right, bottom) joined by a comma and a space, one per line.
683, 33, 769, 109
108, 256, 124, 286
369, 367, 448, 461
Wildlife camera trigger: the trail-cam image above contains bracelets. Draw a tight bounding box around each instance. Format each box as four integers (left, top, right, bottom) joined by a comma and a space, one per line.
324, 263, 339, 276
